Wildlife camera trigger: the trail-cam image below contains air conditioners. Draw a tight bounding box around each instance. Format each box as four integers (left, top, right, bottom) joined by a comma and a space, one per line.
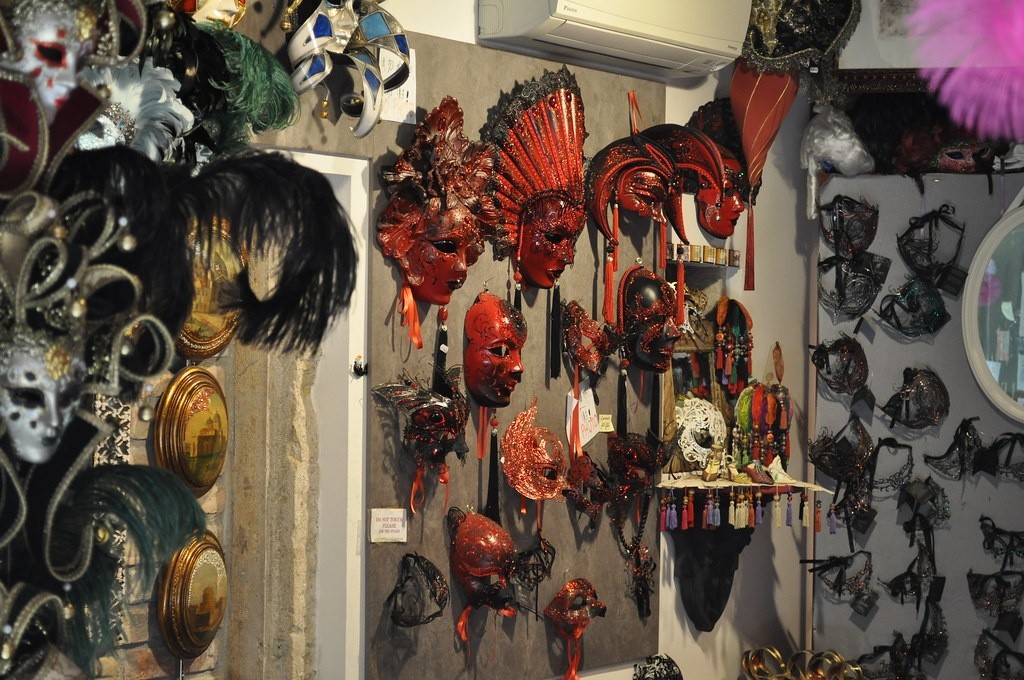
478, 0, 752, 79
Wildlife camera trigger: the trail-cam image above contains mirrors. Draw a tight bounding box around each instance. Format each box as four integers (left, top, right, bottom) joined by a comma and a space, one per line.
958, 182, 1024, 422
